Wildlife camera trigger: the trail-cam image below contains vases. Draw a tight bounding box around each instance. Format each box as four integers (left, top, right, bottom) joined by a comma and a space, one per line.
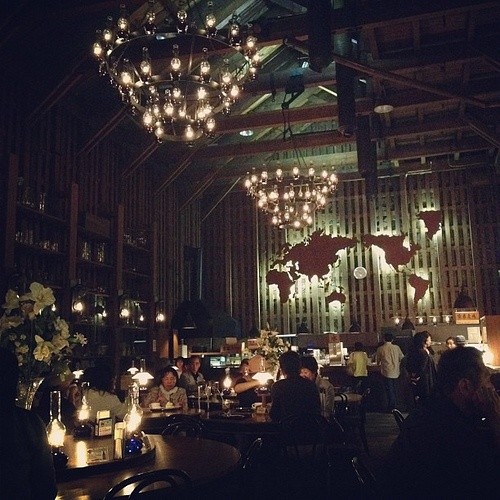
15, 378, 45, 410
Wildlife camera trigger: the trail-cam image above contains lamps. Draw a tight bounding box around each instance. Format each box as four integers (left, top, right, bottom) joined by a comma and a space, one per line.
94, 0, 262, 147
127, 359, 154, 386
372, 94, 396, 114
244, 74, 340, 230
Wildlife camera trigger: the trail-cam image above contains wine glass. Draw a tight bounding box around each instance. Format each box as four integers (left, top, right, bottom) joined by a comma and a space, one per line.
222, 399, 231, 417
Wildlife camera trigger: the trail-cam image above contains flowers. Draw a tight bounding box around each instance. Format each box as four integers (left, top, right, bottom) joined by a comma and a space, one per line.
0, 281, 89, 402
250, 322, 290, 366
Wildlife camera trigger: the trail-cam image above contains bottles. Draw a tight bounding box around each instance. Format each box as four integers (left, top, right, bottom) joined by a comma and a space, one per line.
123, 380, 143, 443
44, 391, 67, 448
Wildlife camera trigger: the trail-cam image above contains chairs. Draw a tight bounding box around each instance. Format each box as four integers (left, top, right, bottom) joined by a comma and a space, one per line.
163, 412, 206, 438
393, 408, 406, 429
106, 467, 193, 500
234, 393, 383, 500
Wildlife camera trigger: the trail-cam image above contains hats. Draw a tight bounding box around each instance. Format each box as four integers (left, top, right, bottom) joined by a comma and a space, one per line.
385, 334, 393, 339
455, 335, 466, 345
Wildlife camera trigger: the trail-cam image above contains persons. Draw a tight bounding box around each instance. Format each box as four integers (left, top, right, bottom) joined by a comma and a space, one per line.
404, 331, 467, 407
376, 334, 405, 413
234, 351, 335, 421
139, 356, 205, 409
0, 348, 58, 500
50, 349, 127, 425
389, 346, 500, 500
347, 342, 369, 394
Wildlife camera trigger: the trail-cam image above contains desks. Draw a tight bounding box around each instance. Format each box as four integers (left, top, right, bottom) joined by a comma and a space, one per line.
335, 392, 363, 432
49, 434, 243, 500
117, 407, 205, 434
189, 393, 235, 408
203, 409, 273, 435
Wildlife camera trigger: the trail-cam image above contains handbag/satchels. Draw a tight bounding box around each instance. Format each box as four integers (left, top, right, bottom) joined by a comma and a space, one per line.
241, 438, 268, 470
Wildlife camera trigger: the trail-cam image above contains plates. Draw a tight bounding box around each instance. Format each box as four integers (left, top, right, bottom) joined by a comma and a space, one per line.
150, 407, 161, 410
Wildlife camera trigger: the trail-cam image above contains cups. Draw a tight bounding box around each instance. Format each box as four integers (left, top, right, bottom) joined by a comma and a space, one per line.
149, 402, 160, 408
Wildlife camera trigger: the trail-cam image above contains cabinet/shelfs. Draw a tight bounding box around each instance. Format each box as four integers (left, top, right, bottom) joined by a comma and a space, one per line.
0, 153, 157, 388
